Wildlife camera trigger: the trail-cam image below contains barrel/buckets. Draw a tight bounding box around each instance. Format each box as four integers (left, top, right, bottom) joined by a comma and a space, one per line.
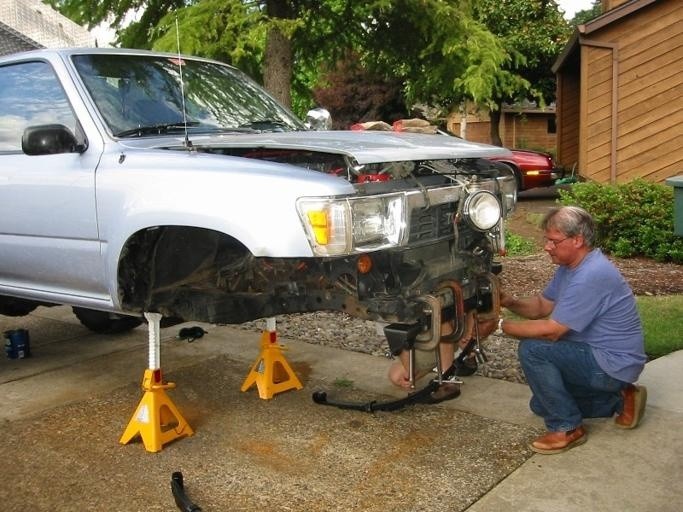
3, 329, 30, 359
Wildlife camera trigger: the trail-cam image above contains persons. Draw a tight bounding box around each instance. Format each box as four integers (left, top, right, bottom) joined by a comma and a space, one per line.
478, 205, 647, 454
389, 311, 497, 392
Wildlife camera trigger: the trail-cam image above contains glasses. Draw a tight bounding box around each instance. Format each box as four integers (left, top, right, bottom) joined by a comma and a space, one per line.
544, 237, 568, 246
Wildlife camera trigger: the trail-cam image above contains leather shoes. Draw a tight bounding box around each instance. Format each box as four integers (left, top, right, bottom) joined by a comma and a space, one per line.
531, 426, 586, 454
615, 387, 647, 429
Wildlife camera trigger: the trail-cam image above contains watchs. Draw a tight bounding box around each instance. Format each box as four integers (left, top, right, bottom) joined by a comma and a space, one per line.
496, 319, 503, 336
